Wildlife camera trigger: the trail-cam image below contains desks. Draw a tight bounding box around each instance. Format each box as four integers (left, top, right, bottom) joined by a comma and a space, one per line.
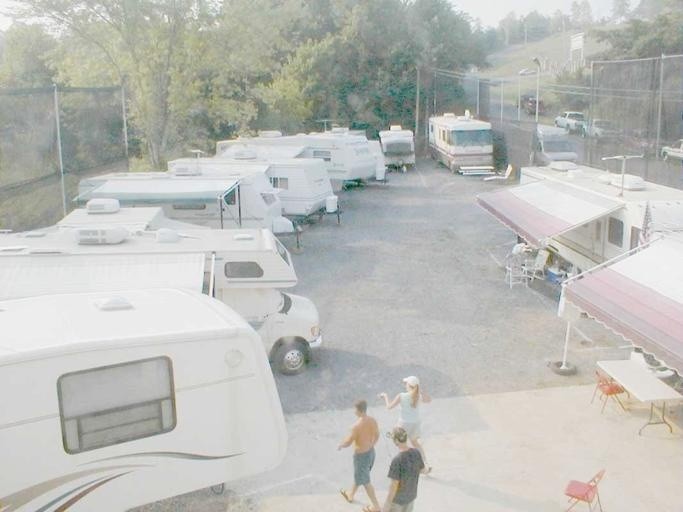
596, 359, 683, 436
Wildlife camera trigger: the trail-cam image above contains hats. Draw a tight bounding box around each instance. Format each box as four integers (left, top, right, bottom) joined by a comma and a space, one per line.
402, 375, 420, 388
385, 427, 407, 441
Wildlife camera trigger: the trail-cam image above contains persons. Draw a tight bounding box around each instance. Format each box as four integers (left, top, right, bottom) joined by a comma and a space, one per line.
382, 425, 431, 512
337, 400, 382, 512
379, 376, 433, 474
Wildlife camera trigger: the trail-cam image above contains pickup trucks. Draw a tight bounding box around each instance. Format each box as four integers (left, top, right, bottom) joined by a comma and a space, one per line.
517, 69, 535, 121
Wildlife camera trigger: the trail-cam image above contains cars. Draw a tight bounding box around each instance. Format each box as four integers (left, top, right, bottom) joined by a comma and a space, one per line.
517, 93, 542, 115
530, 111, 683, 166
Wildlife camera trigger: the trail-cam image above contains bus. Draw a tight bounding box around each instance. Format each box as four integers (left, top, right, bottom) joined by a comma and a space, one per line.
429, 109, 493, 174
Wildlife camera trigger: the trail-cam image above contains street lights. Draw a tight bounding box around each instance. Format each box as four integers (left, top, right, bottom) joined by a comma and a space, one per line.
532, 56, 541, 123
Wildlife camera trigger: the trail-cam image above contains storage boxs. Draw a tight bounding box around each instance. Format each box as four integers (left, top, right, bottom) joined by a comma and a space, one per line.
547, 267, 567, 282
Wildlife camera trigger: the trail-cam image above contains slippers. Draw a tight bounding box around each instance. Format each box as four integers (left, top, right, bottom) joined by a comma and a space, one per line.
362, 504, 381, 511
339, 486, 353, 502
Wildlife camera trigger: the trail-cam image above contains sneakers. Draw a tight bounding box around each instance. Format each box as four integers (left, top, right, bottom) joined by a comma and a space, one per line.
423, 464, 431, 473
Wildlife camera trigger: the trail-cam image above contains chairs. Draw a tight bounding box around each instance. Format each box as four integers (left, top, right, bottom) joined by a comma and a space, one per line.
591, 369, 626, 414
565, 467, 607, 512
505, 248, 550, 289
